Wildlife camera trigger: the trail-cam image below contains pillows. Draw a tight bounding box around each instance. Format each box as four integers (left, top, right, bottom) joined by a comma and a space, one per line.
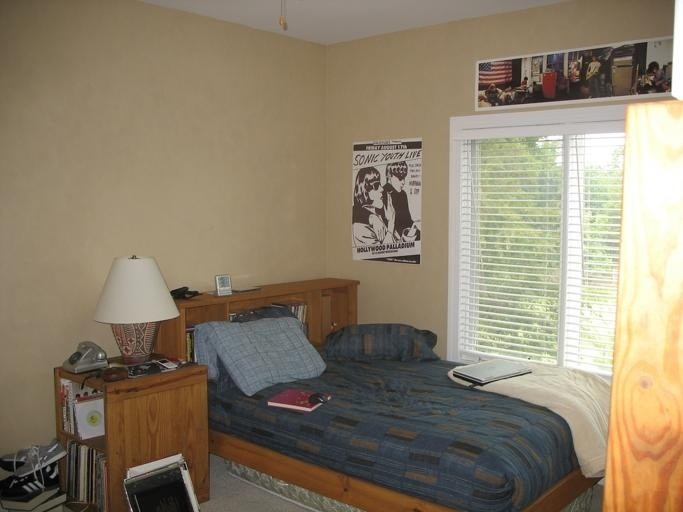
192, 305, 328, 397
324, 320, 442, 365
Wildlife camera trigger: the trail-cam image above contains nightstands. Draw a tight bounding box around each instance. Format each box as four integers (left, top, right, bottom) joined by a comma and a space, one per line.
52, 353, 212, 512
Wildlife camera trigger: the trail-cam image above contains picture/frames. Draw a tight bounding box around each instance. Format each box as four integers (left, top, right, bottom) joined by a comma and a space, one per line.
474, 34, 673, 113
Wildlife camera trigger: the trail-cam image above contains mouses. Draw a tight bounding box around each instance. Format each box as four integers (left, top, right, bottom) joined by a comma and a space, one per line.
308, 393, 326, 403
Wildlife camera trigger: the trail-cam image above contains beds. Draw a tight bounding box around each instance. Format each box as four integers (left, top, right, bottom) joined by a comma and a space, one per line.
152, 274, 610, 511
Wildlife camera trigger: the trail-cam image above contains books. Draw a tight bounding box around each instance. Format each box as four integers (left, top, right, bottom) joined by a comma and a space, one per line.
185, 302, 308, 367
267, 385, 332, 413
58, 377, 108, 511
123, 451, 202, 512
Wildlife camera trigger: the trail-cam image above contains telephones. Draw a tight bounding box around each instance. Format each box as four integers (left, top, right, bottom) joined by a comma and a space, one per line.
63, 341, 108, 374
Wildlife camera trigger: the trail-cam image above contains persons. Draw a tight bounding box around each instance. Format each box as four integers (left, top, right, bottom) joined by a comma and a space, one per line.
352, 166, 401, 261
374, 160, 422, 242
545, 50, 672, 105
485, 83, 502, 106
519, 77, 530, 98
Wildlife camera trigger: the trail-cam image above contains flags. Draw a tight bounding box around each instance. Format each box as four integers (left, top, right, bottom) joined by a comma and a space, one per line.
479, 60, 512, 86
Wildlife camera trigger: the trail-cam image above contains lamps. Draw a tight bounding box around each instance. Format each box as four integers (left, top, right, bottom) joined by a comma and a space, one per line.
93, 252, 180, 364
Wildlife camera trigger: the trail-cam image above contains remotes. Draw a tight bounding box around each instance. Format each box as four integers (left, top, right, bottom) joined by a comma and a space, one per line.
156, 358, 177, 370
